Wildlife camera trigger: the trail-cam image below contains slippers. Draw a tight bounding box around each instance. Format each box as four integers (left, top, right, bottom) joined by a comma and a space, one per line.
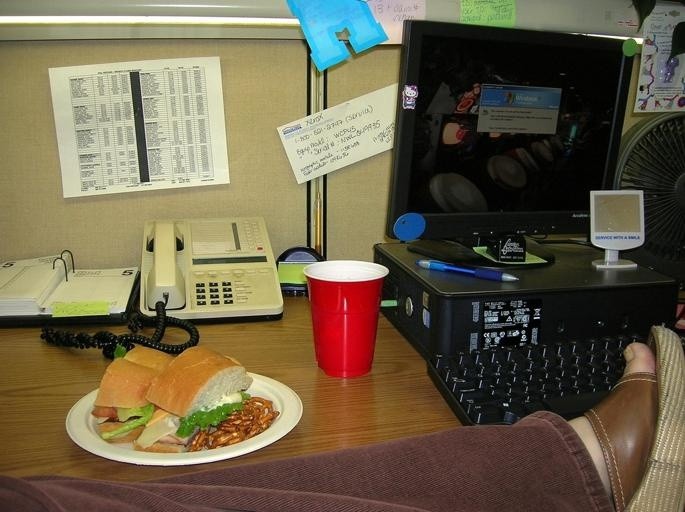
585, 326, 685, 512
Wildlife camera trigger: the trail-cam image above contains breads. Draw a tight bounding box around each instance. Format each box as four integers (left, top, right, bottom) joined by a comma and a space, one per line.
94, 346, 253, 453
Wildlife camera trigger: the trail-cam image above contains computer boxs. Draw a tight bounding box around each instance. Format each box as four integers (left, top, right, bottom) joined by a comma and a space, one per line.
371, 242, 677, 365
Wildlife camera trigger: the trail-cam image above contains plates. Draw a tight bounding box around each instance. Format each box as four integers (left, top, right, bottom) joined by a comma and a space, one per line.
65, 369, 302, 464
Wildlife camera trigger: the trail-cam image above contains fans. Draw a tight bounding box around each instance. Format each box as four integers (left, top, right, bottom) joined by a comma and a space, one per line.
612, 110, 682, 284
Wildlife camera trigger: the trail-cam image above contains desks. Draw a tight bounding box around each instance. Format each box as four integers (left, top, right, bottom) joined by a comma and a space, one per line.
2, 296, 468, 484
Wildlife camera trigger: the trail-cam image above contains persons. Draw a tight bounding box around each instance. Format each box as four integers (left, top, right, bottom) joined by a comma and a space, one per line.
0, 325, 685, 511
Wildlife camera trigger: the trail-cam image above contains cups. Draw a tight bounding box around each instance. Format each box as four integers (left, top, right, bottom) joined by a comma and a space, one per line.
302, 260, 390, 378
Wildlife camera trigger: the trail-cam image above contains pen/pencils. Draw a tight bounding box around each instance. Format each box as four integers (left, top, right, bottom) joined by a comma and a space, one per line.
417, 258, 520, 283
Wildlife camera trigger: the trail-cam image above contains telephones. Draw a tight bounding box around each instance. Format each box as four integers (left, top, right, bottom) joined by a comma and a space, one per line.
139, 217, 284, 325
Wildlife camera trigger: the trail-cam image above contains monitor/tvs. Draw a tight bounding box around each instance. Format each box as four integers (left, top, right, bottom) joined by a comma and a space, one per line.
385, 17, 636, 273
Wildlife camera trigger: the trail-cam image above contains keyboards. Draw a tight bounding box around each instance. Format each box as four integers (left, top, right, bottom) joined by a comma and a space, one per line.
427, 332, 684, 428
